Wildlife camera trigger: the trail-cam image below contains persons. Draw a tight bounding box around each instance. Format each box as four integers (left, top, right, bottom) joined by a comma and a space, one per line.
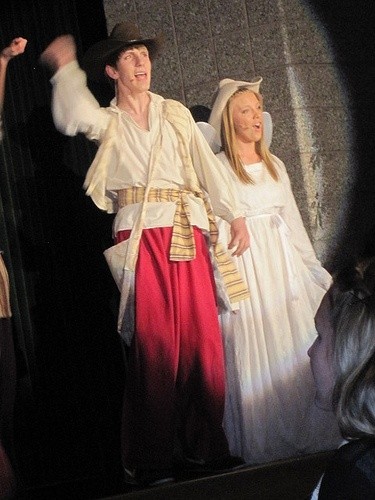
289, 255, 375, 500
191, 72, 350, 466
46, 22, 253, 488
2, 36, 26, 495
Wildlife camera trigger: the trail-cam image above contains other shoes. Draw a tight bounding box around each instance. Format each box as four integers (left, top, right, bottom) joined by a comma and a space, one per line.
121, 466, 175, 485
183, 453, 245, 471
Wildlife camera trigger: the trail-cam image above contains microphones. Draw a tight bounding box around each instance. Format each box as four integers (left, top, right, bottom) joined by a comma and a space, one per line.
232, 122, 247, 129
117, 73, 134, 81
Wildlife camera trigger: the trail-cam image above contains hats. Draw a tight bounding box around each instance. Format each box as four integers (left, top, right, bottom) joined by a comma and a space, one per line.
209, 77, 263, 147
88, 22, 159, 66
